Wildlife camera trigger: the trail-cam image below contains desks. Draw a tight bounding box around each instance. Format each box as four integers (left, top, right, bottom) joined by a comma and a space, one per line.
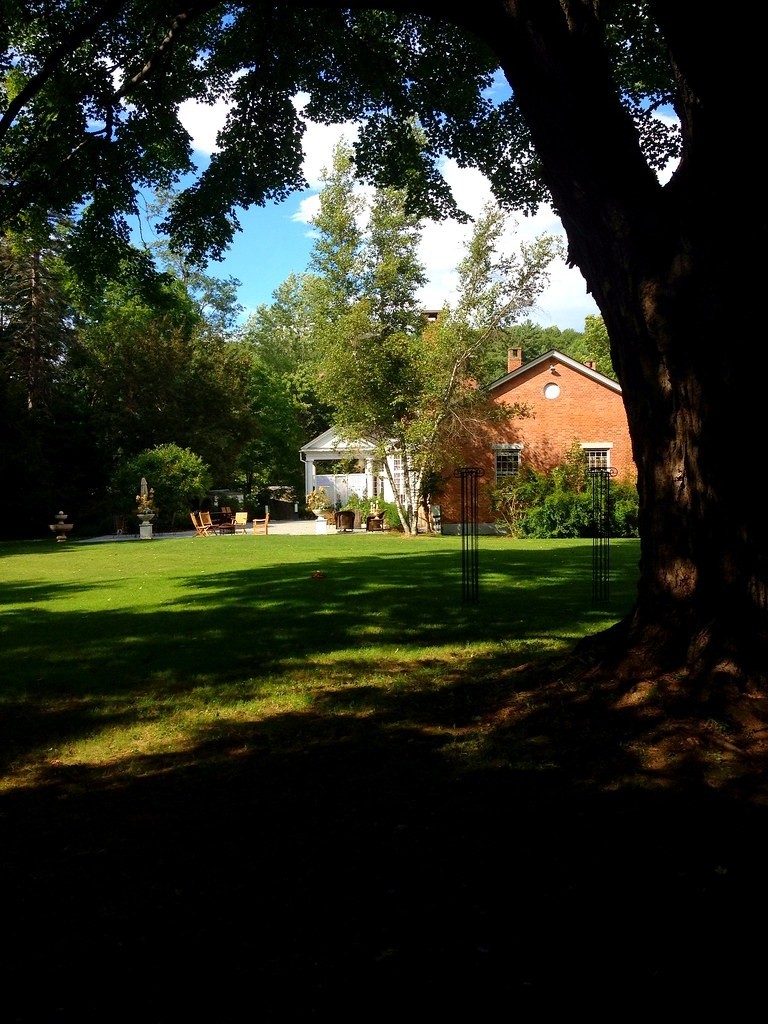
219, 526, 235, 535
211, 512, 233, 523
370, 519, 384, 532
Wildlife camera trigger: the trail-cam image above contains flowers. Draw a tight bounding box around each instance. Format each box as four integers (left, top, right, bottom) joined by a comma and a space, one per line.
304, 486, 328, 511
134, 487, 156, 513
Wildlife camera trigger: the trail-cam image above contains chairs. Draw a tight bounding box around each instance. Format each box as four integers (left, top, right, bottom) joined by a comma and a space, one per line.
226, 507, 232, 516
221, 507, 226, 516
231, 512, 248, 535
252, 513, 270, 535
366, 509, 387, 531
335, 510, 355, 532
199, 511, 219, 536
190, 513, 208, 537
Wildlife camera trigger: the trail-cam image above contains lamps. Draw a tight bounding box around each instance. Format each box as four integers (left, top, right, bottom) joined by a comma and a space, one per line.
549, 365, 557, 373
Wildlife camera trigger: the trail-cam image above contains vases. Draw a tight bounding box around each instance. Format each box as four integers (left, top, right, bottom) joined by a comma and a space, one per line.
312, 509, 327, 519
137, 514, 155, 539
